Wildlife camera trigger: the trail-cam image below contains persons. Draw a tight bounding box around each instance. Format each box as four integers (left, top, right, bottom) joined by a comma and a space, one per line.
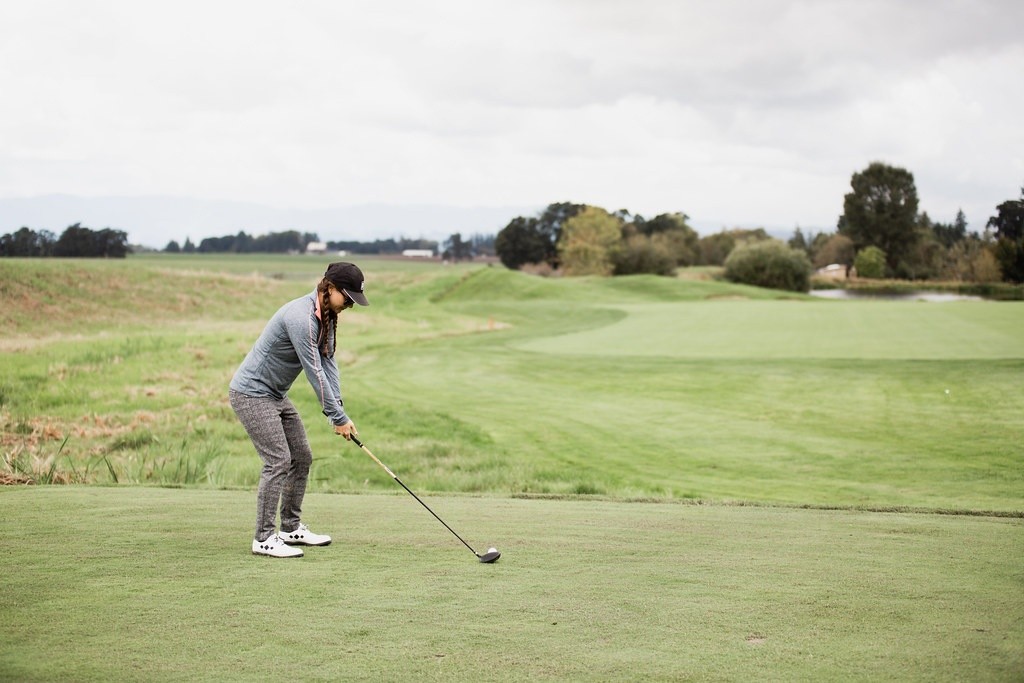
228, 262, 370, 559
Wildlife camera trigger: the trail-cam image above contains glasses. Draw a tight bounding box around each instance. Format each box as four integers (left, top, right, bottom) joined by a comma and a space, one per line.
336, 289, 355, 308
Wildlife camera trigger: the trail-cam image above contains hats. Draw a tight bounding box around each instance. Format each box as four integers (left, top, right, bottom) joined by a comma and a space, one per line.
325, 262, 369, 307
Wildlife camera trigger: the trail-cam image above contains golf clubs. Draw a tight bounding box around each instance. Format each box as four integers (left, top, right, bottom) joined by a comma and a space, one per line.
350, 432, 502, 564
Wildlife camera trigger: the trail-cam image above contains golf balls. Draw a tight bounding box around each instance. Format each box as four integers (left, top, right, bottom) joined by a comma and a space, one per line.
486, 546, 498, 553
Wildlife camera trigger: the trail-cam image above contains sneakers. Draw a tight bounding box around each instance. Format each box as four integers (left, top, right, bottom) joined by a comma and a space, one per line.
279, 523, 331, 546
252, 533, 305, 558
